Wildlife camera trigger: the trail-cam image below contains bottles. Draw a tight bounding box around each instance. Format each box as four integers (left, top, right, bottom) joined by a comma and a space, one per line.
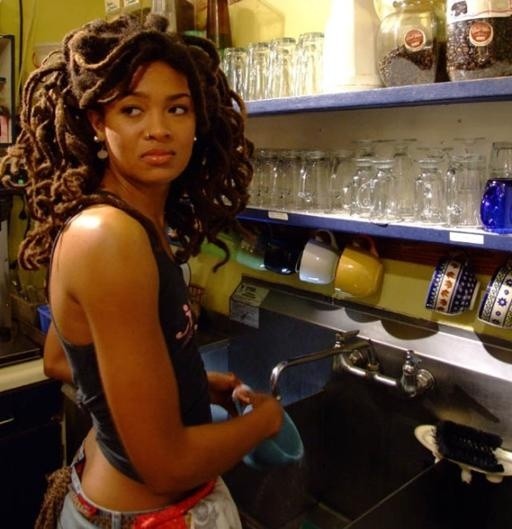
479, 140, 511, 236
240, 146, 351, 212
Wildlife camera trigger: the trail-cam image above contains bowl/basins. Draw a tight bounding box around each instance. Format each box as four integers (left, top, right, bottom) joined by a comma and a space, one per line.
231, 399, 306, 469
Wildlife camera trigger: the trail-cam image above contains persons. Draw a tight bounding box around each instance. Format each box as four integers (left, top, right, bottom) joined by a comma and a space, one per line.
2, 4, 285, 529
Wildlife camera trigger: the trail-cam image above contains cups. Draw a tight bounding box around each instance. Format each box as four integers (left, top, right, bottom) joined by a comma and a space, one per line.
473, 254, 512, 329
263, 228, 300, 276
424, 249, 482, 316
214, 32, 324, 102
296, 229, 342, 285
198, 223, 264, 274
331, 233, 385, 299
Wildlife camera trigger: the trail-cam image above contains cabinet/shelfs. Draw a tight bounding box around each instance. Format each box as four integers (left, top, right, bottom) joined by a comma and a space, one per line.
215, 74, 512, 252
1, 376, 66, 529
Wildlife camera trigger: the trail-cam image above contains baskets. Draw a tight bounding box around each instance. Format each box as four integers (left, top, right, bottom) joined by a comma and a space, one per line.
38, 305, 51, 331
10, 288, 38, 325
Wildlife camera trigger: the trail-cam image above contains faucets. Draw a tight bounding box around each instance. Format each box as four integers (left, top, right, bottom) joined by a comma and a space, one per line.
266, 339, 382, 402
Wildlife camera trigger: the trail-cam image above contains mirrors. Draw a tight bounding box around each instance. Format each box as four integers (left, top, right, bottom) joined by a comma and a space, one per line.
1, 0, 223, 367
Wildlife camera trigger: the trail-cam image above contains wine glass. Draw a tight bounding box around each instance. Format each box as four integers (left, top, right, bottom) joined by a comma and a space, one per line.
344, 135, 485, 229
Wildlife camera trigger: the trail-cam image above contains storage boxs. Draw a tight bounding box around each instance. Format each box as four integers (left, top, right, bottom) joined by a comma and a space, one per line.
37, 304, 53, 332
